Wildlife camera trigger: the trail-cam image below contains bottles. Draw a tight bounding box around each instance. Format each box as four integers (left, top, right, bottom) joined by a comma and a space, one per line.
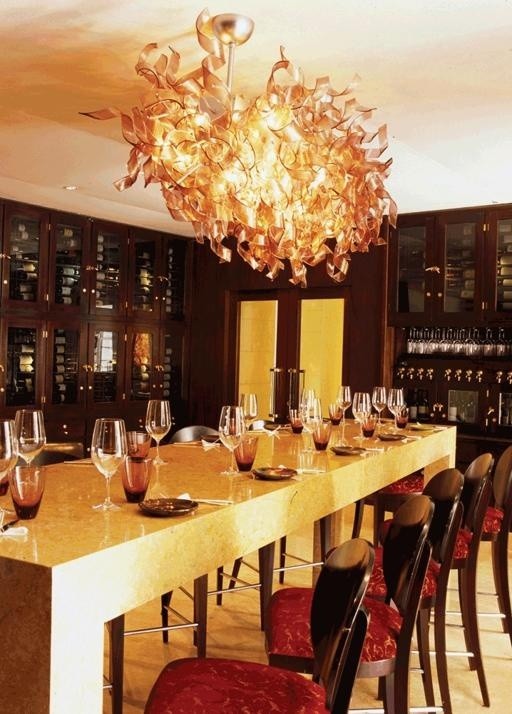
131, 332, 154, 400
54, 328, 78, 404
92, 329, 118, 403
166, 240, 184, 317
8, 326, 36, 405
9, 215, 39, 302
398, 219, 511, 314
135, 236, 154, 313
93, 229, 119, 310
406, 388, 430, 422
163, 331, 183, 401
55, 223, 80, 304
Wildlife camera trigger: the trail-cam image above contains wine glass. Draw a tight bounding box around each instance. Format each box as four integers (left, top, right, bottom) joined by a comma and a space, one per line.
334, 383, 371, 448
146, 397, 173, 466
14, 407, 46, 469
370, 385, 386, 428
386, 387, 404, 433
90, 418, 127, 512
240, 392, 258, 433
403, 327, 511, 358
298, 388, 322, 454
0, 418, 19, 519
217, 405, 243, 476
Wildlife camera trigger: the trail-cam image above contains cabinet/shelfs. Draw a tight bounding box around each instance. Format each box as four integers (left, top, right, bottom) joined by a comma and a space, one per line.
377, 203, 512, 532
1, 198, 196, 466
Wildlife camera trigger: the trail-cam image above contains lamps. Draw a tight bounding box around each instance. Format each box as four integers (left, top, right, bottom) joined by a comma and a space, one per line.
79, 8, 398, 289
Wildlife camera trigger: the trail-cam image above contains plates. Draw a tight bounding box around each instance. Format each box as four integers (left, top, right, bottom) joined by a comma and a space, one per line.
330, 446, 366, 456
138, 496, 199, 515
251, 465, 297, 481
377, 433, 407, 440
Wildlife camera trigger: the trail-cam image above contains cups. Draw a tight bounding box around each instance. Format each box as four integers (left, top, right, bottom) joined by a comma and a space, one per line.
9, 464, 47, 519
328, 403, 343, 425
313, 422, 332, 450
235, 434, 259, 471
289, 408, 304, 433
121, 429, 151, 504
360, 416, 377, 438
